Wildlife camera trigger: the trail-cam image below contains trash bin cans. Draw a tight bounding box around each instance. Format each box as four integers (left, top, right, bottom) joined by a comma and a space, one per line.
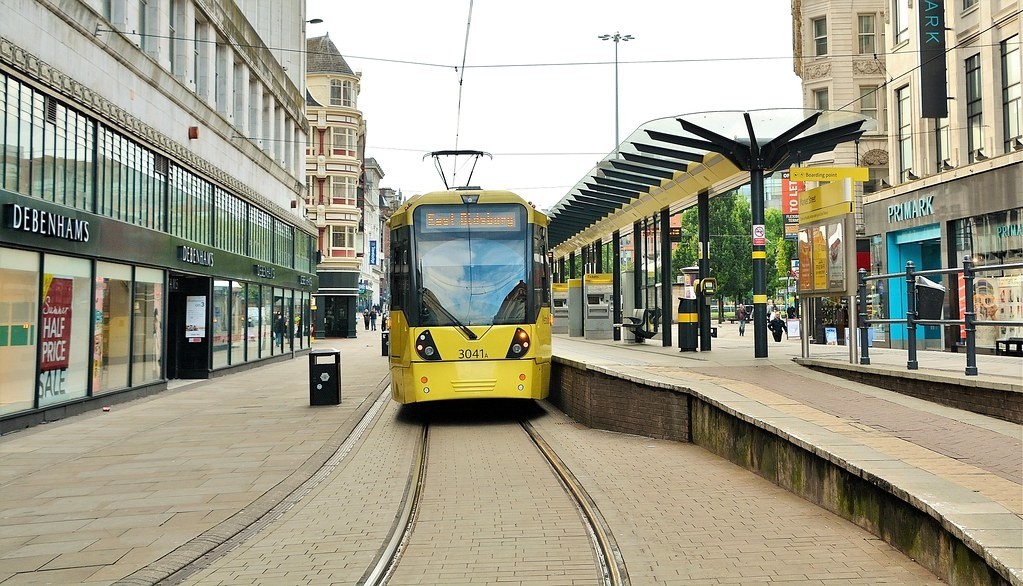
308, 349, 341, 406
677, 297, 699, 352
381, 330, 389, 356
914, 275, 946, 327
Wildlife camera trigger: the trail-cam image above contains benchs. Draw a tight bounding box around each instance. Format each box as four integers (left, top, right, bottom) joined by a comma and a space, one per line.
727, 317, 754, 324
611, 308, 657, 343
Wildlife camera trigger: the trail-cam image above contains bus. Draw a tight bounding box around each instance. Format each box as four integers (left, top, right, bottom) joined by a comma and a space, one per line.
386, 186, 553, 404
711, 304, 785, 320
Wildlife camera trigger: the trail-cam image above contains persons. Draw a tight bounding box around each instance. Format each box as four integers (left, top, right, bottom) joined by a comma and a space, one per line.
736, 303, 749, 336
363, 308, 370, 331
242, 312, 300, 348
373, 303, 381, 316
766, 302, 797, 343
369, 308, 377, 331
